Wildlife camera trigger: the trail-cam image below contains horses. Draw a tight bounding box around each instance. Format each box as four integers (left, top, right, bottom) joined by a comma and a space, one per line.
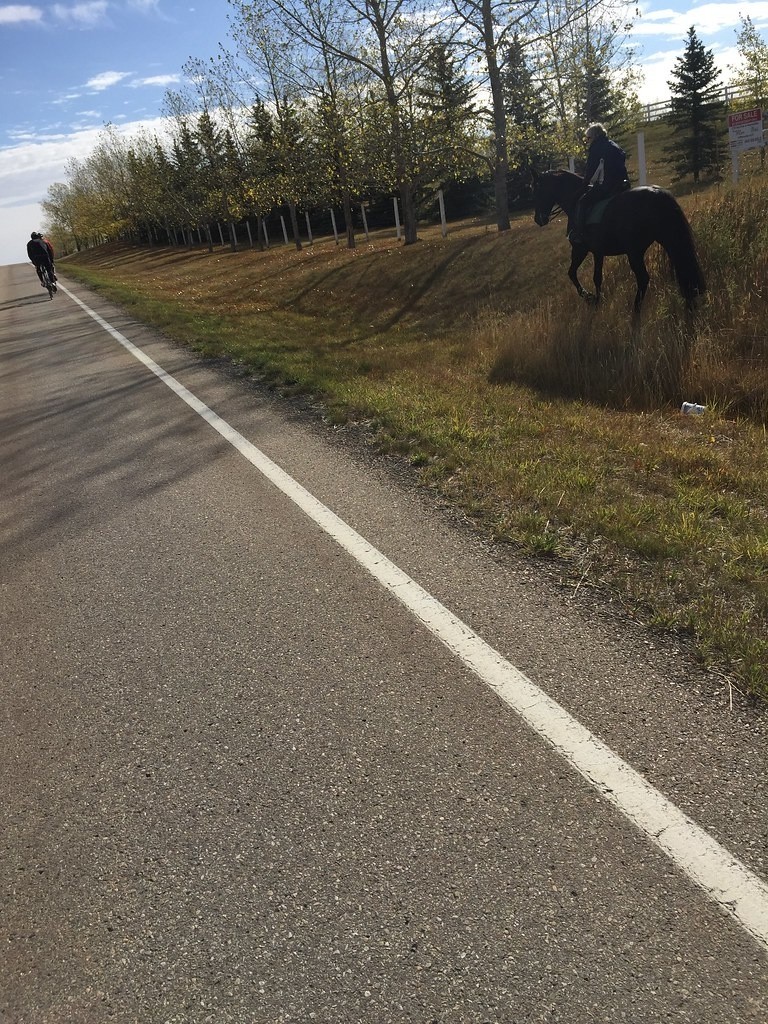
529, 165, 702, 312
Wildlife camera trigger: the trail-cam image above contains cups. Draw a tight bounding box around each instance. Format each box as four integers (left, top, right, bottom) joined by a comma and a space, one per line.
681, 402, 706, 413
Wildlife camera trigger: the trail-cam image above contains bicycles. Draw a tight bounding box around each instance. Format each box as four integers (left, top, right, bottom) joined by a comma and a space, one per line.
34, 260, 57, 300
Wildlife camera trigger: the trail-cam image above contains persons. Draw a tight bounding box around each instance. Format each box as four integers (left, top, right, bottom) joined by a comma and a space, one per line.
27, 231, 58, 292
566, 123, 632, 245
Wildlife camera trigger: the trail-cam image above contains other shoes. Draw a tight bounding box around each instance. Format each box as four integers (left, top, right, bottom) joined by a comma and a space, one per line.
41, 282, 45, 287
51, 283, 57, 291
